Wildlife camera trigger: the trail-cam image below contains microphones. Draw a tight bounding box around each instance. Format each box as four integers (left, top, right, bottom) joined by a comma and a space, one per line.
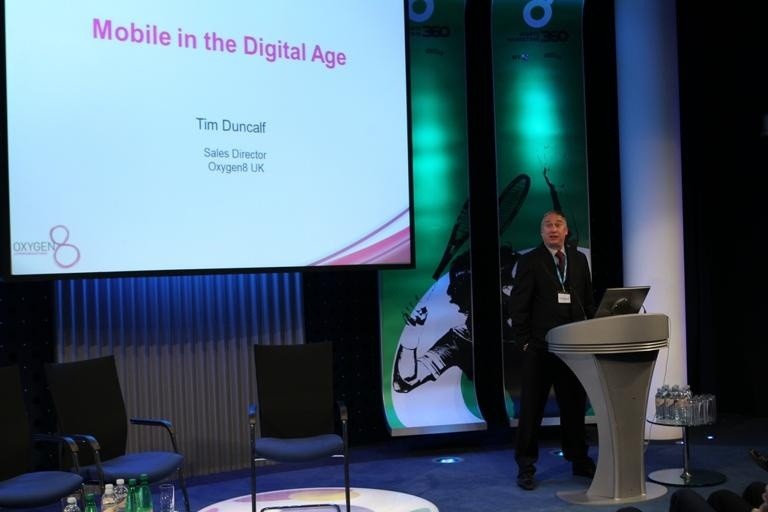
556, 263, 587, 320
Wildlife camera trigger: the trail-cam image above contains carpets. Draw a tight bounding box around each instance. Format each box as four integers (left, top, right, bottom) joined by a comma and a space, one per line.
193, 487, 437, 512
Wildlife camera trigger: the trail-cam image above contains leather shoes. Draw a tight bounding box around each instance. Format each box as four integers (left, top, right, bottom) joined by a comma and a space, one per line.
517, 477, 534, 490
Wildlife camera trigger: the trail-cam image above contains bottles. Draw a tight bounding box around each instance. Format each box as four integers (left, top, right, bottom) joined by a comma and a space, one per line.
63, 471, 154, 511
655, 384, 715, 424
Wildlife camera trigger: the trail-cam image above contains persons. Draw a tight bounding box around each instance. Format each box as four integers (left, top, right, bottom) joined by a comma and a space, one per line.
510, 210, 596, 491
613, 481, 767, 511
749, 447, 767, 474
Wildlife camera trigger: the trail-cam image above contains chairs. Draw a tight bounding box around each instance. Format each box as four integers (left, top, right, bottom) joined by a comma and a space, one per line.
243, 341, 350, 512
41, 355, 191, 512
1, 367, 81, 512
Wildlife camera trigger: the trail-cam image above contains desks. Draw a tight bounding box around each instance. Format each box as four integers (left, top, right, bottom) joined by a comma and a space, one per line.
645, 415, 727, 488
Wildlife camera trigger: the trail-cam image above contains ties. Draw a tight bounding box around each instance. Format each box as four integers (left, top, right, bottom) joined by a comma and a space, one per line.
555, 251, 565, 281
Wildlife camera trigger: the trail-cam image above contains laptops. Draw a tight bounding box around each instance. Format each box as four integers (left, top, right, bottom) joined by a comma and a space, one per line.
594, 286, 650, 318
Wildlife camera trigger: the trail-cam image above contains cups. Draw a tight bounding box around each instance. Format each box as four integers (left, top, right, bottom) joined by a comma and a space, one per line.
157, 483, 176, 512
